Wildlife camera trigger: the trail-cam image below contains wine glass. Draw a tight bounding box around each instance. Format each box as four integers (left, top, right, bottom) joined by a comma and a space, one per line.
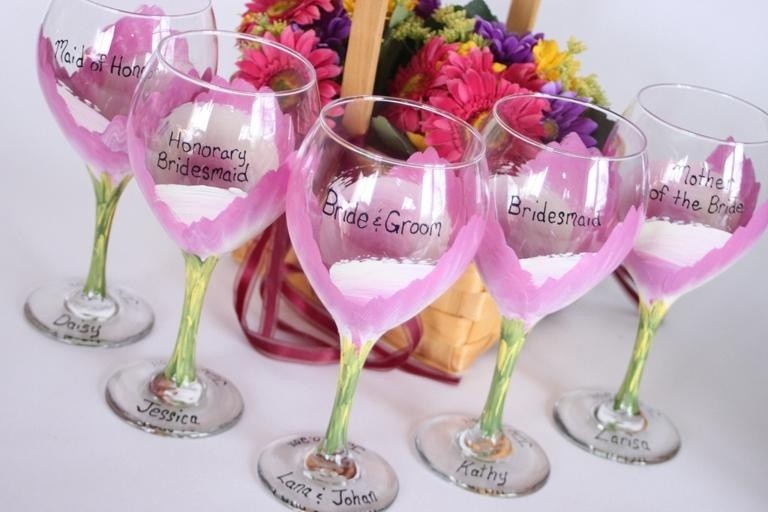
104, 31, 322, 439
24, 0, 218, 348
258, 95, 490, 512
415, 92, 649, 496
551, 83, 767, 464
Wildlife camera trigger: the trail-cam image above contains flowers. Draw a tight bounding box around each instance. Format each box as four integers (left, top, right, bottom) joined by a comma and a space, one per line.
233, 0, 614, 179
